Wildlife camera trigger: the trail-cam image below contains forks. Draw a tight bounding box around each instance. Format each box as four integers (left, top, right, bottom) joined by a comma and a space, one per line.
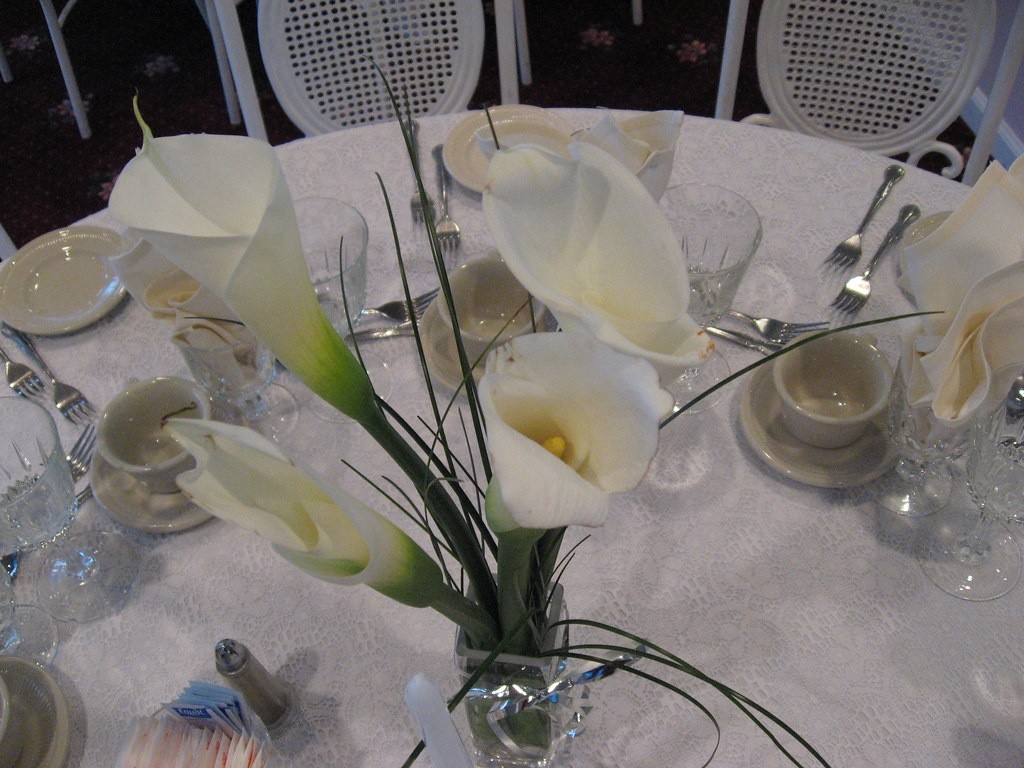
823, 163, 906, 269
361, 287, 440, 322
0, 346, 46, 399
827, 204, 921, 314
402, 120, 436, 224
429, 143, 461, 251
727, 308, 830, 344
0, 322, 97, 425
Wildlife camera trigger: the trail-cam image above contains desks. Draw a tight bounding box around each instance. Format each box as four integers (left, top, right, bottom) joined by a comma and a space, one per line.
0, 111, 1024, 768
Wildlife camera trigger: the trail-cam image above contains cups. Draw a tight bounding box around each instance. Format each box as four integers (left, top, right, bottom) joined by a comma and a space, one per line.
772, 330, 892, 449
435, 256, 548, 369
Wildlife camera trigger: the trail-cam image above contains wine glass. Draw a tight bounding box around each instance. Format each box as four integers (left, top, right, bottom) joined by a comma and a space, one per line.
1, 395, 139, 624
913, 361, 1024, 603
294, 195, 394, 425
658, 182, 763, 415
179, 331, 300, 446
870, 351, 974, 519
0, 563, 60, 671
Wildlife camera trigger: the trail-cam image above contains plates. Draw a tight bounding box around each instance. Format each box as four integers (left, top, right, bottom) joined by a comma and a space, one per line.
738, 357, 899, 489
0, 225, 127, 336
442, 104, 575, 195
419, 296, 558, 397
0, 657, 71, 768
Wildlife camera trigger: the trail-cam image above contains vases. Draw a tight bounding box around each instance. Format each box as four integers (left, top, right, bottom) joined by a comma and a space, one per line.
452, 573, 576, 768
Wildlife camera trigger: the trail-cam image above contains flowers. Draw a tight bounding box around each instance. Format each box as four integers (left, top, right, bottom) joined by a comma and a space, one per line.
110, 97, 840, 768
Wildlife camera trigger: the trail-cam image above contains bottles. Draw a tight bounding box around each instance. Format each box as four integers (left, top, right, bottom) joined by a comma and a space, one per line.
214, 637, 298, 737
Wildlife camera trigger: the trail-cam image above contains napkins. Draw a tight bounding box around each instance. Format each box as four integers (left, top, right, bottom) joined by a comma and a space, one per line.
901, 156, 1024, 427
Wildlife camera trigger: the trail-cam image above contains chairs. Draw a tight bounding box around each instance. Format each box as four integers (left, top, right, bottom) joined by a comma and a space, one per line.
715, 0, 1024, 184
208, 0, 516, 146
